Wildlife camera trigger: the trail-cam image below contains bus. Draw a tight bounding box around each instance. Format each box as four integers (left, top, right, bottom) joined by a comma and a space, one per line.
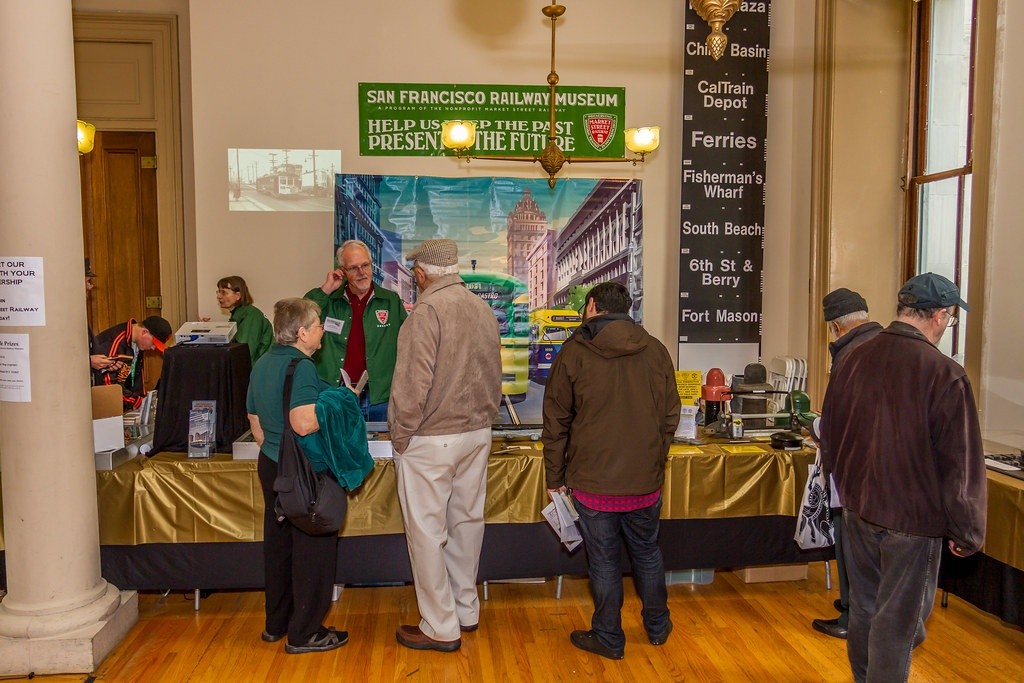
527, 303, 588, 385
414, 270, 528, 401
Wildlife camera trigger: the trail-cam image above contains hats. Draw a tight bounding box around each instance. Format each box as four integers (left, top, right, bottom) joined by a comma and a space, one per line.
85, 256, 97, 277
405, 239, 458, 267
897, 272, 971, 312
822, 288, 868, 321
142, 316, 172, 353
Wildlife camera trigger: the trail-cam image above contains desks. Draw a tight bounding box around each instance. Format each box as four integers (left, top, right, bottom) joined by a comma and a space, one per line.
937, 450, 1024, 627
132, 436, 840, 614
152, 344, 253, 452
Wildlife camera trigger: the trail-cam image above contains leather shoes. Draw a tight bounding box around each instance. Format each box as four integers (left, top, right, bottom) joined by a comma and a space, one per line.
833, 599, 846, 612
395, 625, 461, 651
648, 617, 674, 645
812, 619, 848, 639
570, 629, 624, 660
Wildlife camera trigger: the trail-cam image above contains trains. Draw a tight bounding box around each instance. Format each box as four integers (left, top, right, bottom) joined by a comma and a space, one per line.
254, 173, 302, 199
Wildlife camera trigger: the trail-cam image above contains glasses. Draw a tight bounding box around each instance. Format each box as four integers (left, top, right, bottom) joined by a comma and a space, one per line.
310, 321, 326, 333
216, 290, 235, 296
577, 303, 586, 320
341, 260, 372, 274
945, 310, 958, 327
409, 266, 424, 277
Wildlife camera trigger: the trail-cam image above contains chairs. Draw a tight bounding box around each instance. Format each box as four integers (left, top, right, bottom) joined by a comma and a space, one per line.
768, 355, 808, 423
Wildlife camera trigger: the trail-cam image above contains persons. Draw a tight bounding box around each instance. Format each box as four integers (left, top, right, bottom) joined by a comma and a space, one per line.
91, 316, 173, 414
84, 256, 124, 387
245, 297, 361, 654
817, 272, 987, 683
302, 239, 408, 435
386, 238, 503, 650
540, 282, 682, 659
195, 276, 275, 370
809, 289, 885, 640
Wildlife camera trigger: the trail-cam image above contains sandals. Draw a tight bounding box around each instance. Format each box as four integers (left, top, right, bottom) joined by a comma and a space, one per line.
262, 630, 288, 642
285, 626, 349, 654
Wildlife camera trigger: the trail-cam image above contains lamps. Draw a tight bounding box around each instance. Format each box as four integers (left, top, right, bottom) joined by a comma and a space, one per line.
77, 118, 97, 156
439, 0, 663, 193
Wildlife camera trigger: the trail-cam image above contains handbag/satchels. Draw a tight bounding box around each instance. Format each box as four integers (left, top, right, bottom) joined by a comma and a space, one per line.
272, 426, 348, 536
793, 446, 836, 550
